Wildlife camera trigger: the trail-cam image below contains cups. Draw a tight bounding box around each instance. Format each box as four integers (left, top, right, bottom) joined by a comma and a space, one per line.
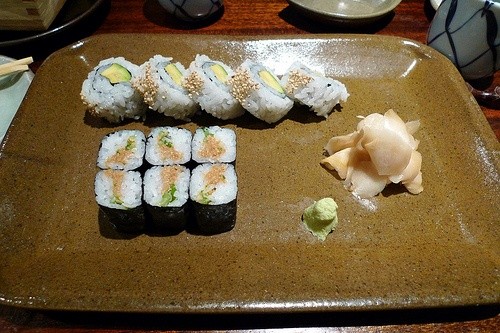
425, 0, 500, 79
158, 0, 224, 20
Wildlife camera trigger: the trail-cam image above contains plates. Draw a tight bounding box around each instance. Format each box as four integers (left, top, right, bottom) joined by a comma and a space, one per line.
0, 0, 102, 51
0, 55, 35, 142
287, 0, 402, 22
0, 33, 500, 311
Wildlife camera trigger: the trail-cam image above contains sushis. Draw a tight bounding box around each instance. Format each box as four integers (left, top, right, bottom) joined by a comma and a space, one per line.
96, 124, 237, 237
80, 53, 351, 124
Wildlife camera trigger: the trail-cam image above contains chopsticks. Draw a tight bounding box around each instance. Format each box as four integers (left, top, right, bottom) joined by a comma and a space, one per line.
0, 55, 34, 76
